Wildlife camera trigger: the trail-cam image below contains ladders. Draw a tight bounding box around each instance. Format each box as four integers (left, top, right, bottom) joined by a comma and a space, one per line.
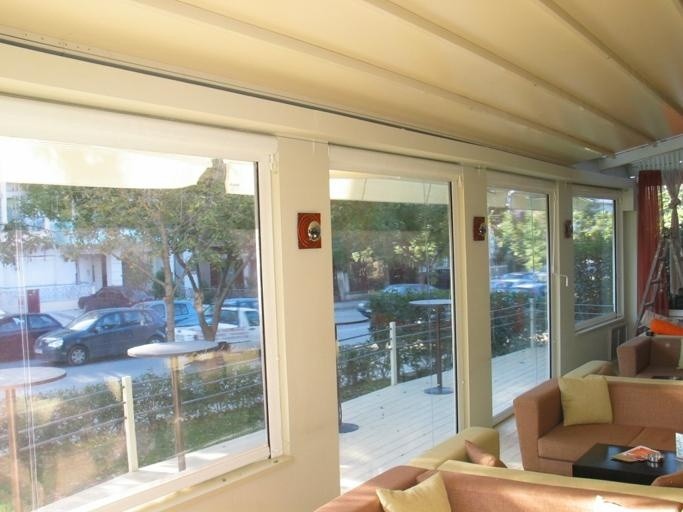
633, 240, 668, 338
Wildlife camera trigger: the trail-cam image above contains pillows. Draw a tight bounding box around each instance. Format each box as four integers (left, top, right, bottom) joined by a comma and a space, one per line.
674, 337, 683, 370
464, 439, 507, 469
649, 467, 683, 488
650, 318, 683, 335
376, 471, 456, 512
556, 373, 615, 427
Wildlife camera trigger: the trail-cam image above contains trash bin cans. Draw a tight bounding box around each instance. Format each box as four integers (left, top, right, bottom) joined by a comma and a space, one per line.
27, 289, 40, 314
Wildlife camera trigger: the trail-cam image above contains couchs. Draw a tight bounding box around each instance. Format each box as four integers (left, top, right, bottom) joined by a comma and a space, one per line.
561, 357, 683, 387
638, 329, 683, 337
513, 369, 683, 478
617, 329, 683, 381
310, 462, 683, 512
409, 420, 683, 501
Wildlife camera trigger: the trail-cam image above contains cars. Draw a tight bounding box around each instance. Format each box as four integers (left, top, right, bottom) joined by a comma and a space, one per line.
490, 270, 547, 300
357, 284, 436, 319
205, 298, 259, 310
32, 307, 165, 367
77, 286, 155, 312
129, 300, 200, 328
0, 313, 66, 363
166, 307, 261, 344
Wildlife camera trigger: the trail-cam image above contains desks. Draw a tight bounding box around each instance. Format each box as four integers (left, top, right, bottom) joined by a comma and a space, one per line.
408, 299, 454, 394
0, 366, 68, 511
334, 316, 371, 435
127, 340, 220, 474
